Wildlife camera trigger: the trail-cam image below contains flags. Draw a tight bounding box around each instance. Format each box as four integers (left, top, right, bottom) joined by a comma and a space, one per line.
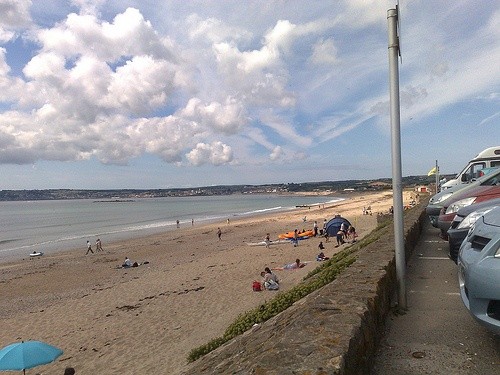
428, 167, 436, 176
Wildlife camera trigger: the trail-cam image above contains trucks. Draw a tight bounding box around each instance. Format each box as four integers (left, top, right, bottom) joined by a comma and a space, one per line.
440, 146, 500, 192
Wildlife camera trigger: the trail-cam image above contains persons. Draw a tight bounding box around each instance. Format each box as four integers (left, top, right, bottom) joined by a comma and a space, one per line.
216, 227, 221, 241
294, 229, 300, 246
314, 221, 318, 237
323, 219, 327, 230
303, 216, 307, 222
64, 367, 75, 375
122, 257, 131, 268
261, 267, 279, 290
265, 233, 270, 248
227, 219, 230, 224
363, 206, 372, 216
96, 239, 104, 253
85, 240, 94, 255
271, 259, 306, 270
336, 221, 355, 246
388, 206, 393, 214
318, 242, 325, 249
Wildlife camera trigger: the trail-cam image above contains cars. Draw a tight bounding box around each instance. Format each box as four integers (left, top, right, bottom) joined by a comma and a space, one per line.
456, 204, 500, 337
437, 183, 500, 242
476, 166, 498, 180
447, 197, 500, 266
426, 167, 500, 228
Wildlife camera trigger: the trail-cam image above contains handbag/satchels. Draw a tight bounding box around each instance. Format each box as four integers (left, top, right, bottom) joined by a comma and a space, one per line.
252, 279, 261, 292
265, 280, 279, 290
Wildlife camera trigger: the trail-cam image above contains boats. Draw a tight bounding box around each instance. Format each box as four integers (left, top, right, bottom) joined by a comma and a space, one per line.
278, 230, 313, 239
242, 239, 292, 247
29, 252, 44, 257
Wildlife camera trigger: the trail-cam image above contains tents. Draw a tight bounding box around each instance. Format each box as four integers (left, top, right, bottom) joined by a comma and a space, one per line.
326, 215, 353, 236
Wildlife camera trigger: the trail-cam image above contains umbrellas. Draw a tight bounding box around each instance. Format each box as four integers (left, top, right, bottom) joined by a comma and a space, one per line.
0, 341, 63, 375
